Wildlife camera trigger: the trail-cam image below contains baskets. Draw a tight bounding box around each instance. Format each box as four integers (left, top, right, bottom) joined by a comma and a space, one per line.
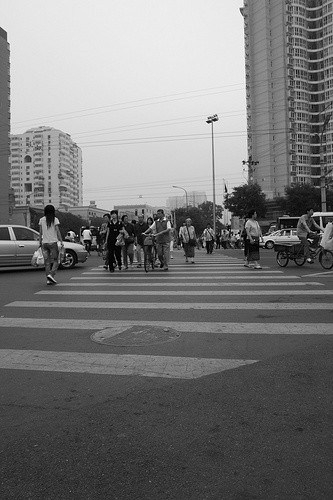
142, 238, 154, 245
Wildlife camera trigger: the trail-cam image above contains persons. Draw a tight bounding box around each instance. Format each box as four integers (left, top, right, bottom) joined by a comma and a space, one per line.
96, 210, 175, 273
82, 226, 93, 250
203, 223, 215, 254
65, 229, 76, 241
179, 217, 196, 263
39, 205, 62, 285
216, 229, 241, 249
296, 207, 324, 264
243, 210, 265, 269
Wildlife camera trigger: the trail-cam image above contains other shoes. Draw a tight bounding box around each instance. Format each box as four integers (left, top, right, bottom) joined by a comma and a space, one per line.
254, 266, 262, 269
307, 260, 314, 263
103, 266, 108, 270
309, 246, 316, 251
244, 264, 252, 268
160, 263, 164, 268
47, 275, 57, 285
192, 262, 194, 264
164, 268, 168, 272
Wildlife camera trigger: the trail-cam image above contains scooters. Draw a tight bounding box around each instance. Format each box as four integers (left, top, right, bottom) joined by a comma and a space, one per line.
63, 234, 79, 243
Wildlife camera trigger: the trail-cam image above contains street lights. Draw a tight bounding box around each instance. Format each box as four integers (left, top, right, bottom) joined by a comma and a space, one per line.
172, 186, 189, 214
25, 189, 40, 227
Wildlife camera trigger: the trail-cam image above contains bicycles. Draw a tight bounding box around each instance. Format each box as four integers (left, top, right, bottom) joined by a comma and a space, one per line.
140, 233, 164, 273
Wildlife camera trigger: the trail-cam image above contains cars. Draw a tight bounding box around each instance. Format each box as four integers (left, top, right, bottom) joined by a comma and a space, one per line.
0, 224, 90, 270
258, 227, 314, 249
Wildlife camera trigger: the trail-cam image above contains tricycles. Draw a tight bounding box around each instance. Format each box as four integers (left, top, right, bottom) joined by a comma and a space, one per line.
273, 231, 333, 270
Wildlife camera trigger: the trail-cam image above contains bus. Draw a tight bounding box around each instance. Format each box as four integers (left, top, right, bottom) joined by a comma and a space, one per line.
310, 211, 333, 234
79, 225, 107, 251
277, 215, 301, 230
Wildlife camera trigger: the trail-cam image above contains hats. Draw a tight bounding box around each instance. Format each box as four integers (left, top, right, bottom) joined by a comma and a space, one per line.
186, 218, 192, 222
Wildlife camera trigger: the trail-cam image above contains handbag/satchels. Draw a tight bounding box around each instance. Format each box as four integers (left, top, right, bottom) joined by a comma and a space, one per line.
189, 239, 197, 247
212, 236, 214, 243
31, 247, 39, 267
126, 236, 135, 243
37, 247, 45, 265
115, 233, 125, 246
251, 236, 259, 245
